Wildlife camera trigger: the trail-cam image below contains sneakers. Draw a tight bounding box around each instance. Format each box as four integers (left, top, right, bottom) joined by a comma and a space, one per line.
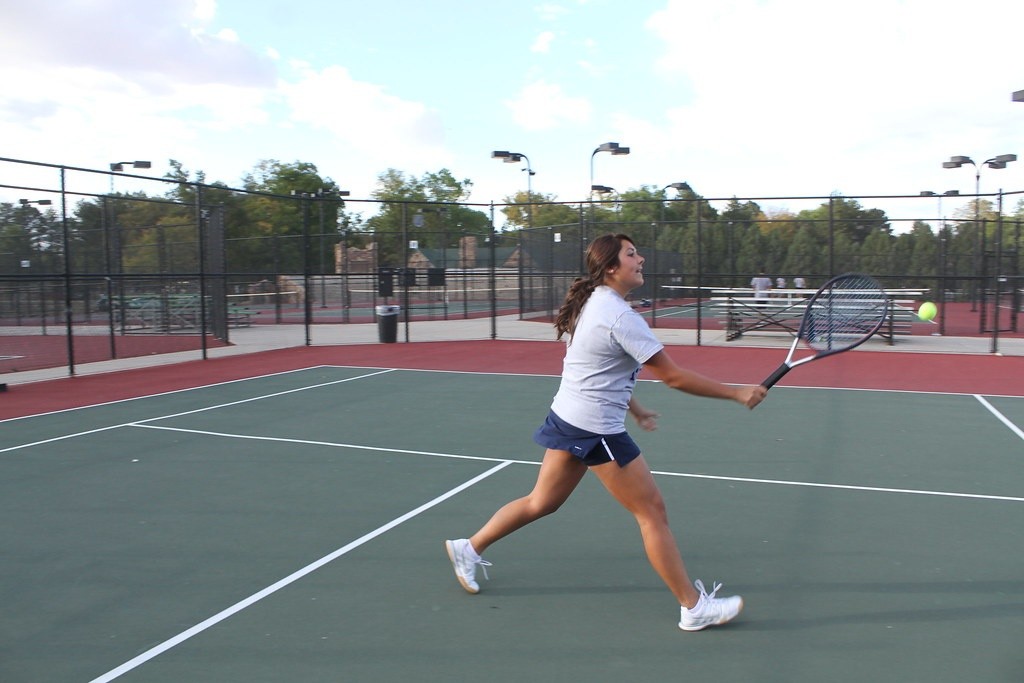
678, 579, 744, 632
445, 538, 491, 593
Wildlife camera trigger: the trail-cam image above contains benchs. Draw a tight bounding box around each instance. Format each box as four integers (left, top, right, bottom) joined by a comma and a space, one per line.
109, 294, 261, 330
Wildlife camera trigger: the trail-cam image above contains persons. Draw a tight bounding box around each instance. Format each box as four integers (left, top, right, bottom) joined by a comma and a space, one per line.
775, 275, 789, 302
444, 231, 770, 630
794, 274, 806, 307
751, 267, 773, 327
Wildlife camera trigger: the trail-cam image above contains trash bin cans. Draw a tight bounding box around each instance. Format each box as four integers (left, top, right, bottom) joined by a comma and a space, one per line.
374, 304, 401, 342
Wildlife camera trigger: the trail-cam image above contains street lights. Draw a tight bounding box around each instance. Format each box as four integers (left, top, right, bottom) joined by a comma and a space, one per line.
589, 141, 630, 201
491, 150, 532, 203
19, 198, 51, 233
416, 207, 449, 233
659, 183, 692, 200
109, 160, 152, 197
920, 154, 1017, 313
291, 186, 350, 308
591, 184, 621, 201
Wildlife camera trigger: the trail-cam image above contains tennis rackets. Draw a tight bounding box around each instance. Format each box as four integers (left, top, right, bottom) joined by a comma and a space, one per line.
758, 270, 888, 392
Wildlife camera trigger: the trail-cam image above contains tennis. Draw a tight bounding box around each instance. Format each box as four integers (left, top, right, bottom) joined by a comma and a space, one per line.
918, 301, 938, 321
